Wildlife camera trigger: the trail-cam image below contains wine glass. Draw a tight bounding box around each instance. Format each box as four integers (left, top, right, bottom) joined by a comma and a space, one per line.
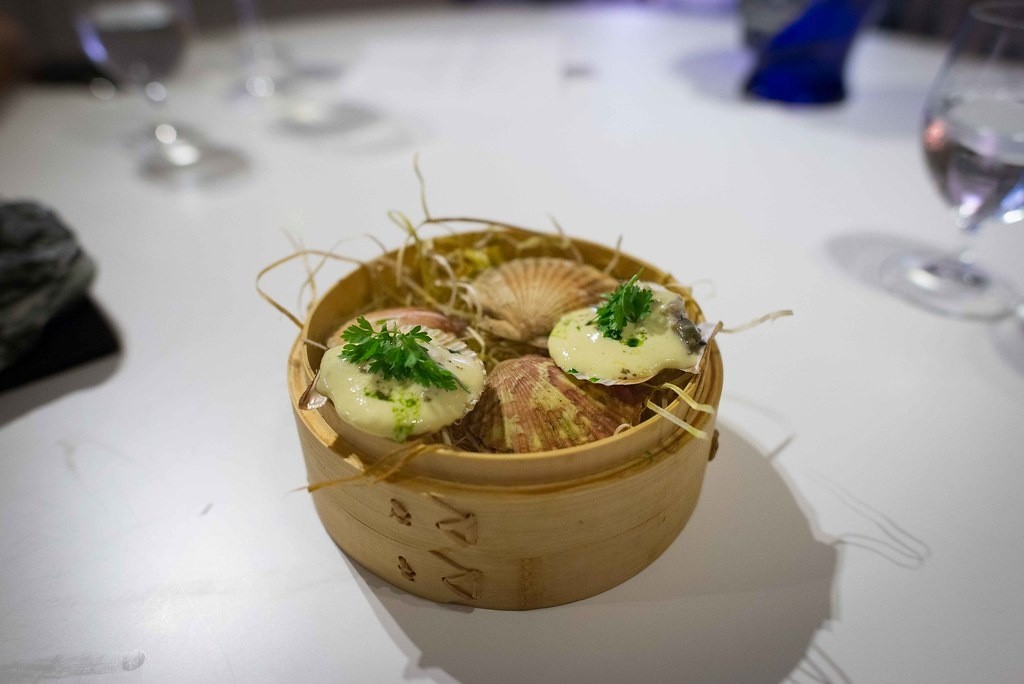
66, 1, 242, 180
880, 3, 1024, 323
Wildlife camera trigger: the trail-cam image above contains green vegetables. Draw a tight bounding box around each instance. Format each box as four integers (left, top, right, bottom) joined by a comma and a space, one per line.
339, 316, 460, 393
582, 264, 655, 343
